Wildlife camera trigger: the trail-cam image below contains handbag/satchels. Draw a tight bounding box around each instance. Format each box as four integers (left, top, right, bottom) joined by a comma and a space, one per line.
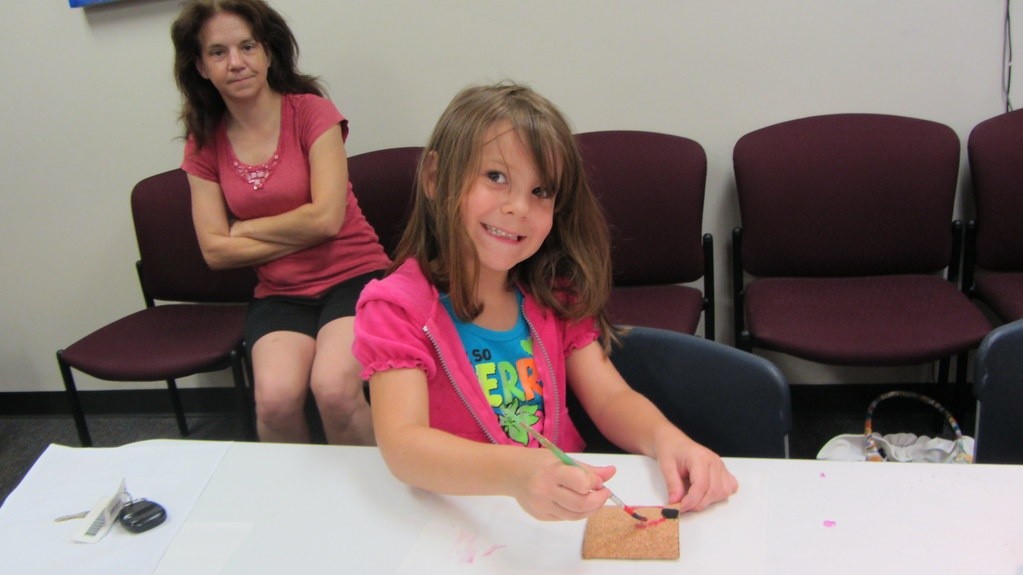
816, 390, 974, 464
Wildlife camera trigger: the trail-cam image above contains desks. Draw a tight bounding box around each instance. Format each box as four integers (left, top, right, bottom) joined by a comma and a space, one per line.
114, 439, 1022, 573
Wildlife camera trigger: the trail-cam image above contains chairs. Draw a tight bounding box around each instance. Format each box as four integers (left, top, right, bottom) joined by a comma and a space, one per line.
728, 111, 986, 429
972, 317, 1023, 464
57, 164, 264, 454
964, 109, 1023, 388
567, 325, 793, 460
345, 148, 444, 264
568, 128, 718, 341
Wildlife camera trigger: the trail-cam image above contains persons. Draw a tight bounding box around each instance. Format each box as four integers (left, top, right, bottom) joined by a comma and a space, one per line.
170, 0, 394, 446
350, 77, 738, 522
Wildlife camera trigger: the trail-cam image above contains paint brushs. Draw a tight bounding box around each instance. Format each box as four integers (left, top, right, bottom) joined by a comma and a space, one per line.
502, 409, 647, 522
